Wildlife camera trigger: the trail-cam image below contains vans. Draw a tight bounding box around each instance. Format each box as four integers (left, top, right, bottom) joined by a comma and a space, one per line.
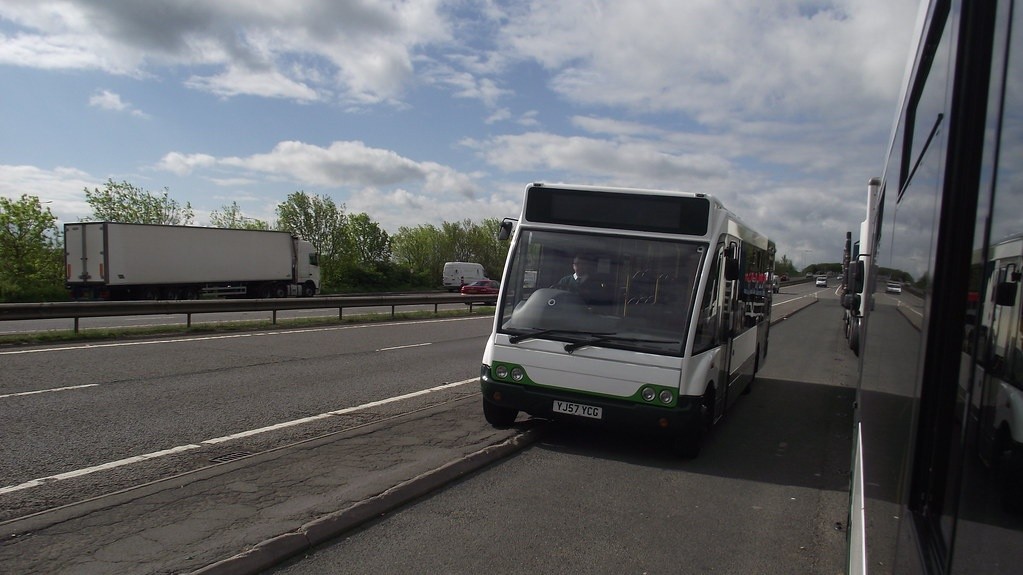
816, 275, 827, 287
773, 275, 780, 294
887, 280, 902, 296
443, 262, 489, 290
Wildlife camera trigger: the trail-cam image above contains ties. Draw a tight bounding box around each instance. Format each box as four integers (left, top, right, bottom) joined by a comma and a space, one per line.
576, 278, 580, 285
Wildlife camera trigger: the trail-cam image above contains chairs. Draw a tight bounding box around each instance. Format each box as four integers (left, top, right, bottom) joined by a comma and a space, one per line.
586, 272, 679, 315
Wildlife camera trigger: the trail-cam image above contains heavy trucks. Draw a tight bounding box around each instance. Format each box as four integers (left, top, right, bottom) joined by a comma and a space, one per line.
64, 222, 323, 297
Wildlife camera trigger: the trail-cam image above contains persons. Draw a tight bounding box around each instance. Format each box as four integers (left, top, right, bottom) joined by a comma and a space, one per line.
555, 255, 598, 311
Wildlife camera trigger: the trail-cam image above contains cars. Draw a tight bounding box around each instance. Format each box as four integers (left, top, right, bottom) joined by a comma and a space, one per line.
837, 274, 844, 279
461, 279, 513, 305
782, 275, 789, 282
806, 272, 813, 278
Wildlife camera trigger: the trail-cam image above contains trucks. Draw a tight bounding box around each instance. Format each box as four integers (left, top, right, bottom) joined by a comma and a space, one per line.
841, 177, 881, 354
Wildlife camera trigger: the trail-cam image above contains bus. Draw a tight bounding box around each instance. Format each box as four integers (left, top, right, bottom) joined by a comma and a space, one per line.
847, 2, 1023, 575
480, 181, 777, 451
969, 236, 1023, 377
